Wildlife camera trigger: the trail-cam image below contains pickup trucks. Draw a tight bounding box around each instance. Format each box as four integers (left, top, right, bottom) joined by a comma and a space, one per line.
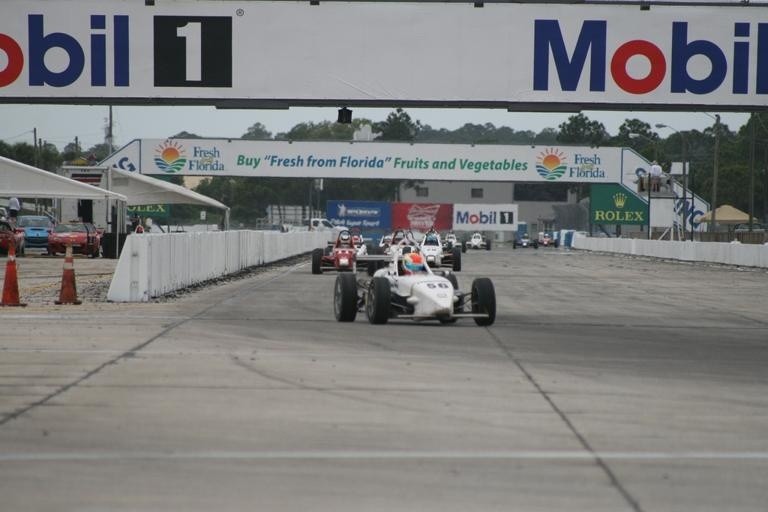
280, 217, 351, 241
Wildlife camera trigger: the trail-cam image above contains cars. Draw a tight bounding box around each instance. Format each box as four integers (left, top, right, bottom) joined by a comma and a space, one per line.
731, 222, 768, 232
0, 206, 104, 262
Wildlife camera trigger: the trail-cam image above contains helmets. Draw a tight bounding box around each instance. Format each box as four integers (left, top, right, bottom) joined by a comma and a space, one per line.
402, 254, 424, 274
384, 236, 391, 244
340, 232, 351, 244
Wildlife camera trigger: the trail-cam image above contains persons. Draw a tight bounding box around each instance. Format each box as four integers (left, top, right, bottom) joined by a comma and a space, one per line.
650, 160, 662, 192
7, 198, 20, 223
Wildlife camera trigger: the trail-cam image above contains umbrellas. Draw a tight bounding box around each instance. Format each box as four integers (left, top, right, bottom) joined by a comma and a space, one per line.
697, 203, 757, 232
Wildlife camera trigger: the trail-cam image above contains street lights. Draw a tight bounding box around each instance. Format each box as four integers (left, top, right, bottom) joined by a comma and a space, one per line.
654, 122, 696, 241
629, 131, 679, 236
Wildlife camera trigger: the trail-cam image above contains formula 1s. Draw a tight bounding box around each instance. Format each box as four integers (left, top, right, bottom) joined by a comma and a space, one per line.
513, 237, 538, 249
330, 239, 499, 326
535, 238, 560, 246
310, 227, 494, 273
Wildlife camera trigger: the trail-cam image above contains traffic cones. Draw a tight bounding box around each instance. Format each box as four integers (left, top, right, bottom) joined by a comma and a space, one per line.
50, 240, 85, 304
1, 239, 28, 307
